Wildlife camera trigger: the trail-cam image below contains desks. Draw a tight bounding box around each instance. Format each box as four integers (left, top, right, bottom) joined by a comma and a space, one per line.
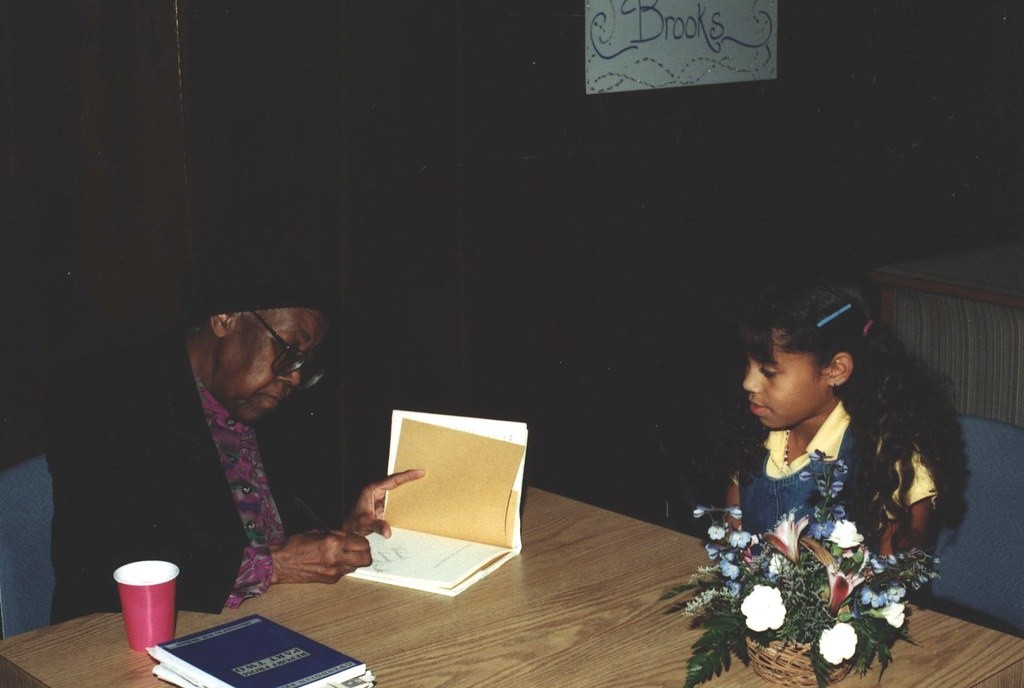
0, 483, 1024, 688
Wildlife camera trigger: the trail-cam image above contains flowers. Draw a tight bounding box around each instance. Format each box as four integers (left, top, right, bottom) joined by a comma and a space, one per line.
660, 450, 944, 688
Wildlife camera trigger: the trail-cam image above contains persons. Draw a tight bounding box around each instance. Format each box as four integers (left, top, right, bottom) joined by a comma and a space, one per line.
49, 237, 425, 618
722, 268, 935, 597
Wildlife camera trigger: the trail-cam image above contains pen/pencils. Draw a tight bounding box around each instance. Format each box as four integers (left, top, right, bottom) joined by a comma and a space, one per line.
295, 497, 331, 534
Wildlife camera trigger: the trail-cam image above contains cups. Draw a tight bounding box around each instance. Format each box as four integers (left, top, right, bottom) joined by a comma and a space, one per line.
113, 560, 179, 650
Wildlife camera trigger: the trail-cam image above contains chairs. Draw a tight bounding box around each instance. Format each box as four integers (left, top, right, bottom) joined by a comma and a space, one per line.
907, 411, 1024, 636
0, 453, 57, 639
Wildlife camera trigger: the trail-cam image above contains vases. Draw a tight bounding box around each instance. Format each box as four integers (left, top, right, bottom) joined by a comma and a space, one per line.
745, 633, 859, 688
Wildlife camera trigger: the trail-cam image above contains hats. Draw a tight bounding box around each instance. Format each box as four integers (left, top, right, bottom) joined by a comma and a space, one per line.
185, 244, 344, 328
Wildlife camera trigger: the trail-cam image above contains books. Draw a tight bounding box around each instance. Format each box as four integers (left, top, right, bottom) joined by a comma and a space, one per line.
151, 612, 367, 687
345, 407, 530, 597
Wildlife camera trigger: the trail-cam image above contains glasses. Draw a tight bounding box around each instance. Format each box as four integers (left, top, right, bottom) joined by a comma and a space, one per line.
249, 311, 325, 390
